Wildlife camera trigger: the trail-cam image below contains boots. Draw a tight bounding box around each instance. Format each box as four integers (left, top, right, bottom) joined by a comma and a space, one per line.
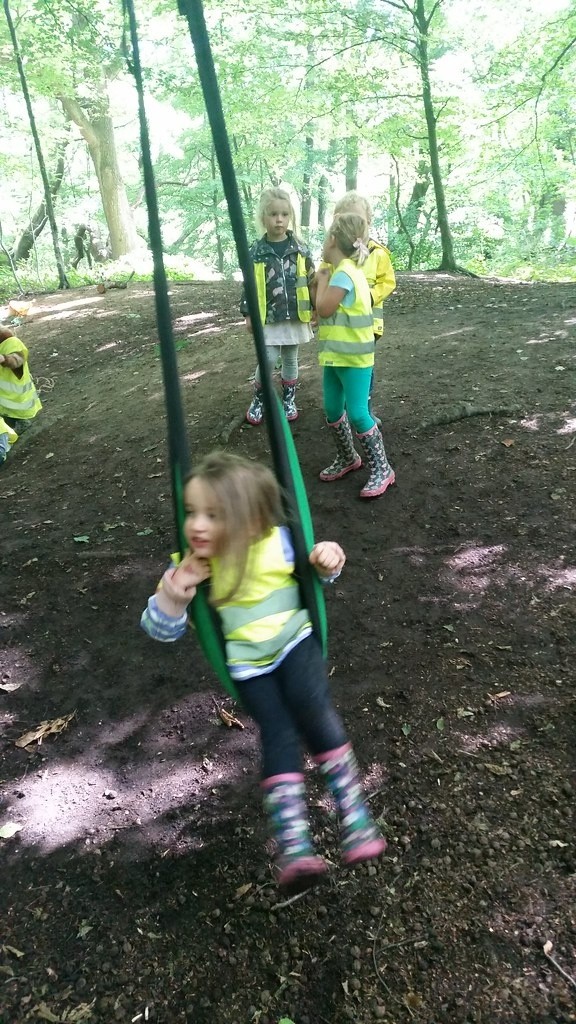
260, 772, 327, 883
320, 411, 362, 481
312, 740, 385, 863
368, 396, 382, 426
356, 422, 396, 496
281, 379, 298, 420
247, 381, 265, 425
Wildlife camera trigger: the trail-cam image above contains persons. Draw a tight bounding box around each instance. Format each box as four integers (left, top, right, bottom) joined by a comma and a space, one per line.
318, 187, 399, 497
139, 447, 388, 891
0, 324, 44, 463
239, 185, 316, 426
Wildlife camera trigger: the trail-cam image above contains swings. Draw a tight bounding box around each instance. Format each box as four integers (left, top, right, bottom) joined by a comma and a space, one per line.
125, 0, 332, 704
